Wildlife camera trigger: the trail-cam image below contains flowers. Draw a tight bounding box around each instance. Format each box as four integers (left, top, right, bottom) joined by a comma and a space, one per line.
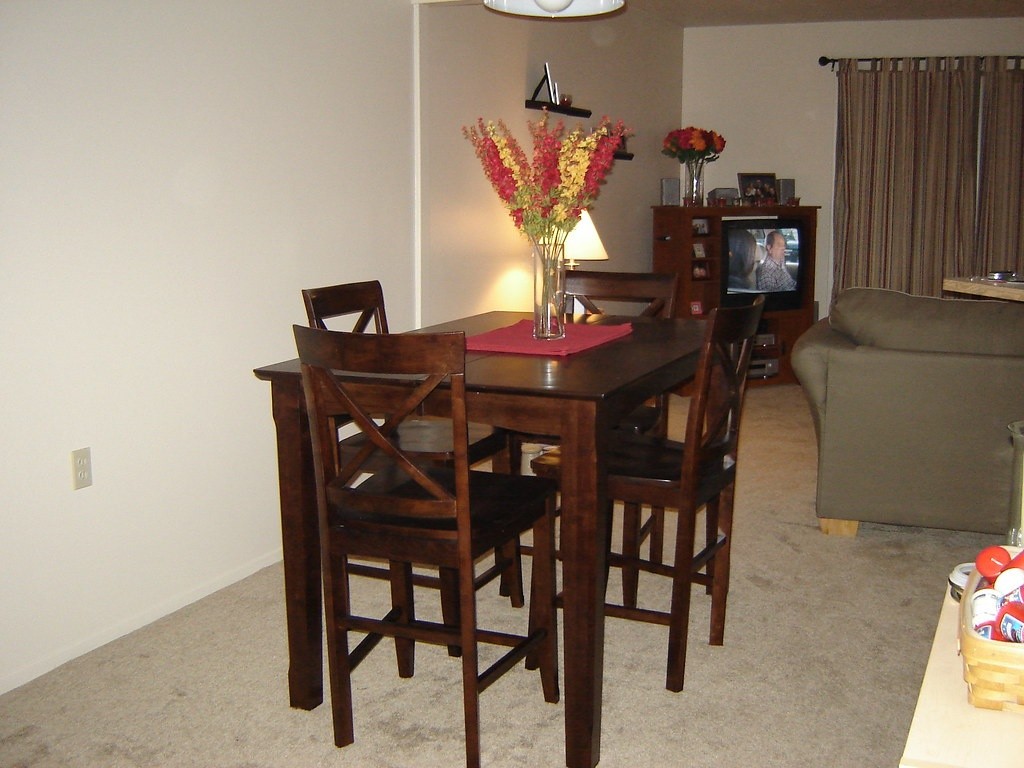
660, 126, 726, 198
461, 106, 633, 336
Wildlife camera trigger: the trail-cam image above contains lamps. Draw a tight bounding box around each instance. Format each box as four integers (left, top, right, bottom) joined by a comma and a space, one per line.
482, 0, 625, 18
531, 209, 609, 271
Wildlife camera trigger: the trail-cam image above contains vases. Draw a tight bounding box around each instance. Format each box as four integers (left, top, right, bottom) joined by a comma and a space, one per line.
528, 244, 566, 340
686, 157, 704, 207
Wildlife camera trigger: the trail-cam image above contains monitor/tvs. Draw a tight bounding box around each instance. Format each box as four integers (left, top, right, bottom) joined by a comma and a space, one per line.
720, 218, 804, 311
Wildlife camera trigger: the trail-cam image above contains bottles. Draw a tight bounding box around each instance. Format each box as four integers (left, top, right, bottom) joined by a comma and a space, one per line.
560, 94, 572, 107
1008, 420, 1024, 547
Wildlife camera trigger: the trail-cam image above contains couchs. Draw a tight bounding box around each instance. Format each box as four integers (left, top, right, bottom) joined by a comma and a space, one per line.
791, 287, 1024, 538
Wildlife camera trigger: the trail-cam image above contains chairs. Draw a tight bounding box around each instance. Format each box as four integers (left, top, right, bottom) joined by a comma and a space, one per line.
291, 271, 766, 768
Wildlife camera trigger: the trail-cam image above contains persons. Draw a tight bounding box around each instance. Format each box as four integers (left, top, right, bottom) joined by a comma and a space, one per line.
745, 181, 776, 206
728, 230, 796, 291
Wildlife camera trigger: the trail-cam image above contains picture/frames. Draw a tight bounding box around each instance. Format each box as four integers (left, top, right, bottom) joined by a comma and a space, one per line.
737, 173, 781, 207
690, 301, 702, 315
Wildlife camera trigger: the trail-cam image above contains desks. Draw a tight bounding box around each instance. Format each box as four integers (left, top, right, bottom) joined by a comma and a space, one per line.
251, 311, 710, 768
900, 583, 1024, 768
942, 277, 1024, 301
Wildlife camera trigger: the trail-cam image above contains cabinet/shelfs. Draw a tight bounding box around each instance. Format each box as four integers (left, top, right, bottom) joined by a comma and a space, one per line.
525, 98, 636, 160
650, 205, 822, 397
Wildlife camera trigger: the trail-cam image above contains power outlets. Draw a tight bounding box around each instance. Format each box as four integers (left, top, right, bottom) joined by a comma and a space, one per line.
70, 446, 93, 490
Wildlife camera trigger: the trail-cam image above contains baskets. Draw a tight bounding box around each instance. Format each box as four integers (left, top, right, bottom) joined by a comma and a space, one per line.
957, 546, 1024, 715
